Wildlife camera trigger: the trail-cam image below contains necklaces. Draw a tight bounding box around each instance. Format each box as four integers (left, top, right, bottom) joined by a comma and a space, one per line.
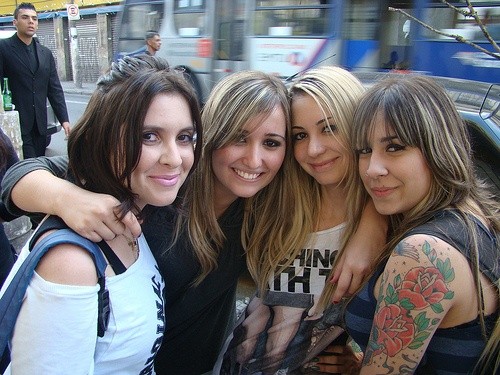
125, 229, 137, 251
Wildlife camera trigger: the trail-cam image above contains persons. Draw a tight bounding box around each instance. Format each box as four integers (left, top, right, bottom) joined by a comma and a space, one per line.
0, 2, 72, 159
144, 32, 161, 56
351, 75, 500, 375
0, 127, 21, 289
1, 71, 388, 375
212, 66, 368, 375
0, 54, 203, 375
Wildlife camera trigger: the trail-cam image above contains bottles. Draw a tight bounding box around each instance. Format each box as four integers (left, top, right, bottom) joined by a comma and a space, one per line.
2, 78, 12, 111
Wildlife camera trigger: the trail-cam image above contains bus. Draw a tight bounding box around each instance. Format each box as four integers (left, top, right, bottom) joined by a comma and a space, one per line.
112, 2, 500, 190
